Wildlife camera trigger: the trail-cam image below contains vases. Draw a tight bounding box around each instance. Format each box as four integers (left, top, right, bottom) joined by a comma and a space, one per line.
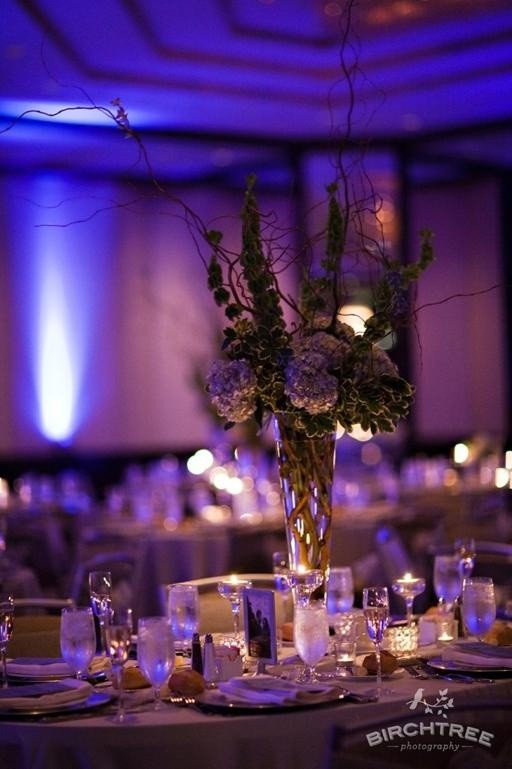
272, 413, 337, 590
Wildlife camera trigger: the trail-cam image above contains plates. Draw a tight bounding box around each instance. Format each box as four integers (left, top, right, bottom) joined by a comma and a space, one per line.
425, 657, 511, 676
0, 655, 113, 718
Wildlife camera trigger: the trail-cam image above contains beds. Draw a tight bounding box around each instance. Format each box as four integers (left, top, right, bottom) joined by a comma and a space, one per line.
0, 616, 510, 767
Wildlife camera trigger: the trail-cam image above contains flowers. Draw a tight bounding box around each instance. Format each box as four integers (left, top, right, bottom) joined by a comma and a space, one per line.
0, 0, 511, 566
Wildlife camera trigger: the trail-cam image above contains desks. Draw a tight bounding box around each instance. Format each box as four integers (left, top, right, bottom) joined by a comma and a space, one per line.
0, 465, 511, 609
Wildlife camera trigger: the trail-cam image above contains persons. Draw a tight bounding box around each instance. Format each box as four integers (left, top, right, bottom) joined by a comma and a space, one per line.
254, 609, 272, 658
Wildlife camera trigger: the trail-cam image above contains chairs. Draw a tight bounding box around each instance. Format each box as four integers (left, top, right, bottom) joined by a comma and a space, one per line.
327, 705, 507, 768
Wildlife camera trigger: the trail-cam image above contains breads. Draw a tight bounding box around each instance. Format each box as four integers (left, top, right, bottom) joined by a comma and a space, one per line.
168, 667, 204, 696
123, 666, 150, 689
282, 623, 293, 641
362, 650, 397, 673
484, 623, 512, 646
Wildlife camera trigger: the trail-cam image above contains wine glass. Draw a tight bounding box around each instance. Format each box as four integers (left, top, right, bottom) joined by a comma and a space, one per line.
0, 592, 17, 689
60, 537, 497, 723
